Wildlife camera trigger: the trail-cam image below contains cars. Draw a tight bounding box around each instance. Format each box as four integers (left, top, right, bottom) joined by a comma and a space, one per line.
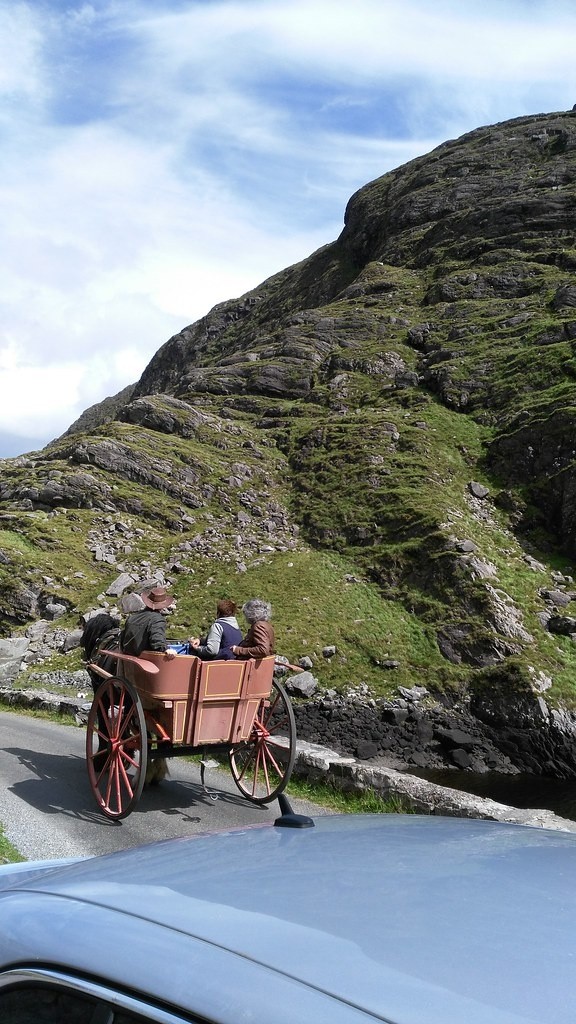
0, 810, 576, 1024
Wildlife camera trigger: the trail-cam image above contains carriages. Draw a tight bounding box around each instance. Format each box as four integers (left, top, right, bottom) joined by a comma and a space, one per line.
76, 614, 297, 818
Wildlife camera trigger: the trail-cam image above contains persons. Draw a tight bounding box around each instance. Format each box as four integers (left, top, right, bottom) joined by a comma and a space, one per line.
123, 588, 178, 659
188, 600, 243, 661
230, 599, 274, 660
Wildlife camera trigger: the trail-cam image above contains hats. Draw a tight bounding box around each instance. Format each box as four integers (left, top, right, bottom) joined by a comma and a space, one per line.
141, 587, 174, 609
